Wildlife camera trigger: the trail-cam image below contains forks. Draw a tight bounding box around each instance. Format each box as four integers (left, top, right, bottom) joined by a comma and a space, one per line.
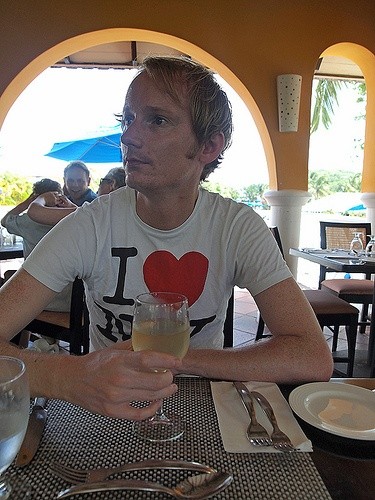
233, 382, 302, 452
50, 460, 216, 484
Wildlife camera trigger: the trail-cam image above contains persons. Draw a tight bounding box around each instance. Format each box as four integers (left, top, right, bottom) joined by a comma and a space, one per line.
0, 161, 126, 346
0, 57, 332, 422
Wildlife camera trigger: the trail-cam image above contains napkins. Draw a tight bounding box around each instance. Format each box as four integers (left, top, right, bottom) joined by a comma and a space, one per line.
210, 380, 314, 454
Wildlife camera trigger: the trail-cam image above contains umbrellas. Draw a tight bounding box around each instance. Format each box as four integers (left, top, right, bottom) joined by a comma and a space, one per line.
43, 123, 123, 163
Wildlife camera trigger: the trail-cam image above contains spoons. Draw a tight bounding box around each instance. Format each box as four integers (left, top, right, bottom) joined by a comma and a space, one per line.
57, 471, 233, 500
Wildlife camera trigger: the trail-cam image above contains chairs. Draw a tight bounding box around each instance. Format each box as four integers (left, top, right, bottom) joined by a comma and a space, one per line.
10, 222, 375, 378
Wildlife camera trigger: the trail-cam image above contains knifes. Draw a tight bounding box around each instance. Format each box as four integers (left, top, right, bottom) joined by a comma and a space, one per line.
16, 396, 49, 467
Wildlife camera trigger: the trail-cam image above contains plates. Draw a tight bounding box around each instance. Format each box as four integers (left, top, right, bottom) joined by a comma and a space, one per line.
288, 381, 375, 441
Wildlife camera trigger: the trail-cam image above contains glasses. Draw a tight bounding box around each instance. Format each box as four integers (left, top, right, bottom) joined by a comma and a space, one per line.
99, 178, 122, 188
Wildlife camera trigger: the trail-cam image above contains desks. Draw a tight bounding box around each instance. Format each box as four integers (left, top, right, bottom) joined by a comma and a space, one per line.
289, 248, 375, 334
1, 355, 375, 500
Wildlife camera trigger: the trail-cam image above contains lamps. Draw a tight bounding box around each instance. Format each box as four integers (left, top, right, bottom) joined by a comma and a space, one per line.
276, 74, 303, 132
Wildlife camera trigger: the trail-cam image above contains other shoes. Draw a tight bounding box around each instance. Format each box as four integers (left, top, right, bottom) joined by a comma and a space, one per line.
34, 339, 59, 353
24, 341, 42, 354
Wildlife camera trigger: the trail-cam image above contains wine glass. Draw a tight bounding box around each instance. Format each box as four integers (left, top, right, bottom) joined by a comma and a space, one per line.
350, 232, 375, 258
131, 292, 190, 441
0, 356, 31, 500
